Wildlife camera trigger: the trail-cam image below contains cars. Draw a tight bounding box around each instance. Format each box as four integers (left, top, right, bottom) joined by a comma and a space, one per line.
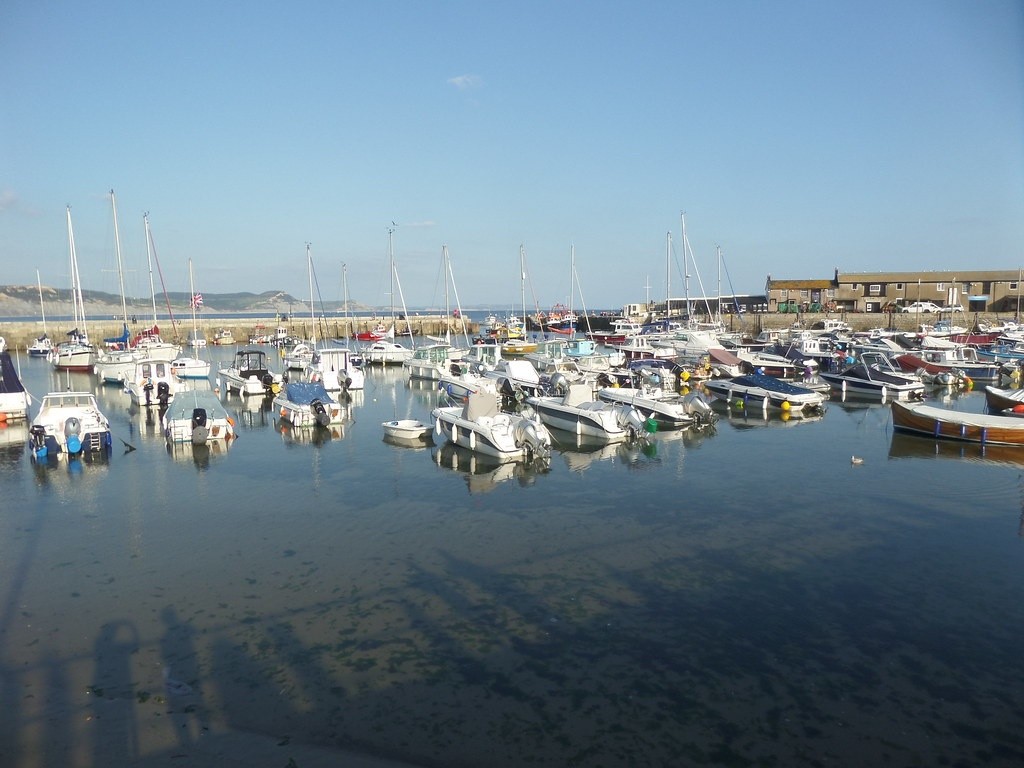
937, 304, 963, 313
902, 303, 941, 313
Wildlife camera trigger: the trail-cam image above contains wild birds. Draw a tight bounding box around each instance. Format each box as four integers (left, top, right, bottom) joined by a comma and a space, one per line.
808, 277, 812, 280
849, 455, 865, 464
841, 270, 887, 273
922, 268, 953, 272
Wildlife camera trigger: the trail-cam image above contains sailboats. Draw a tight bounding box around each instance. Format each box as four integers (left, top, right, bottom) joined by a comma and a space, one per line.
547, 245, 612, 373
606, 212, 772, 360
403, 244, 482, 379
502, 247, 601, 387
26, 188, 416, 382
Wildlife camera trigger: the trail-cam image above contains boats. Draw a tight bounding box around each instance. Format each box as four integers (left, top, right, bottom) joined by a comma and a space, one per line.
525, 385, 656, 449
439, 342, 543, 400
431, 391, 554, 461
26, 368, 110, 455
773, 320, 1024, 411
384, 417, 430, 439
188, 330, 206, 345
704, 370, 824, 410
890, 399, 1024, 446
600, 384, 717, 432
481, 300, 578, 341
272, 381, 347, 425
217, 347, 283, 396
164, 387, 236, 445
